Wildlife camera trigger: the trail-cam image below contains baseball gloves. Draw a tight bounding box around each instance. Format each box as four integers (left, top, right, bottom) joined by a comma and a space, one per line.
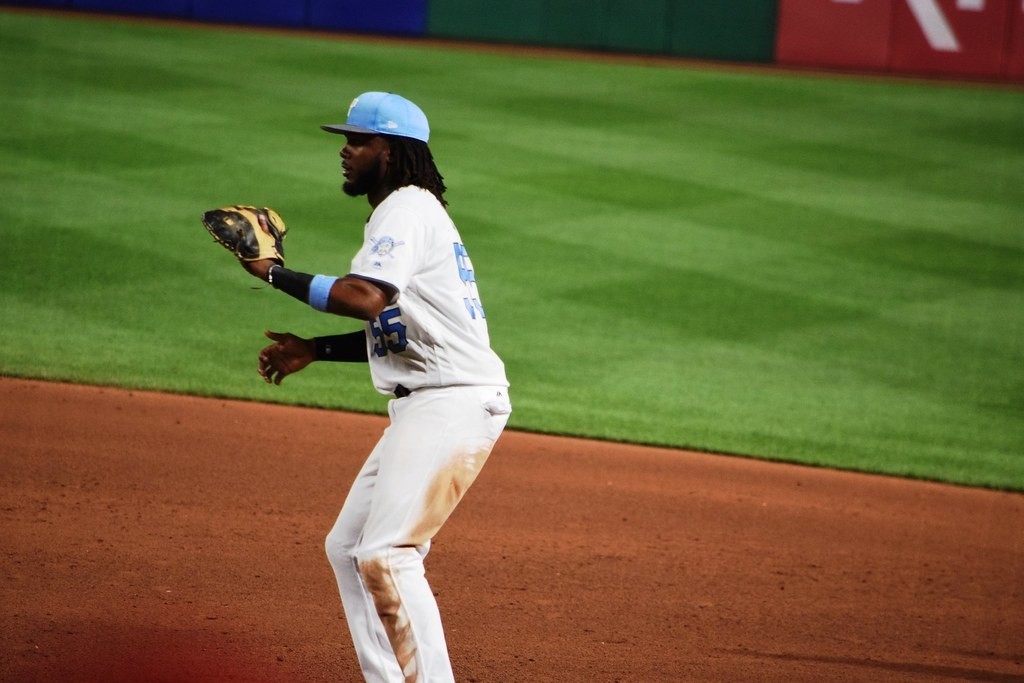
199, 205, 289, 268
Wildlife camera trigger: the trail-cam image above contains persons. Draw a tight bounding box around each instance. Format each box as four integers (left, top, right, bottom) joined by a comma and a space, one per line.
200, 91, 513, 683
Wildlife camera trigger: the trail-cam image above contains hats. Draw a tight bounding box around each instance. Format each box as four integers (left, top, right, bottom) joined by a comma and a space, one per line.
320, 91, 430, 143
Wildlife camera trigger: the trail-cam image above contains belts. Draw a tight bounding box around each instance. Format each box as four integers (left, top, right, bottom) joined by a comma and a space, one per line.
395, 384, 410, 399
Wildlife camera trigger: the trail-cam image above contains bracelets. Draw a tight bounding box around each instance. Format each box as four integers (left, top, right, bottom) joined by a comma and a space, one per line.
326, 336, 331, 361
268, 264, 282, 289
